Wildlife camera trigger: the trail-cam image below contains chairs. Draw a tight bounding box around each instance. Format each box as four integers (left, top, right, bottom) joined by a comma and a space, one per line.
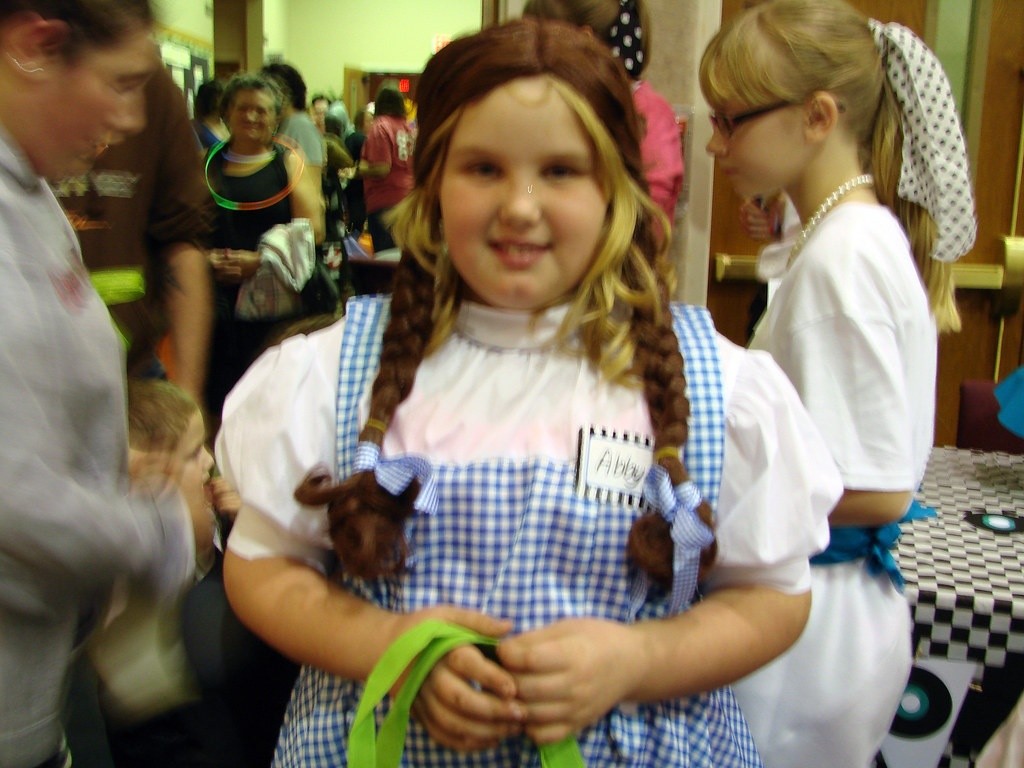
956, 378, 1024, 455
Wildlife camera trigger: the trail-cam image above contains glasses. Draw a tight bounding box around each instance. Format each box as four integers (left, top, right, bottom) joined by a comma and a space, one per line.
708, 98, 791, 141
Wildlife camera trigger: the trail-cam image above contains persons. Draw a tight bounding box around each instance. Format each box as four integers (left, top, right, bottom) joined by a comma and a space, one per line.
526, 0, 974, 768
213, 19, 845, 768
0, 0, 421, 768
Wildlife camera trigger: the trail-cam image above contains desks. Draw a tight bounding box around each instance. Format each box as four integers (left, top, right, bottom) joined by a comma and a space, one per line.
890, 444, 1024, 757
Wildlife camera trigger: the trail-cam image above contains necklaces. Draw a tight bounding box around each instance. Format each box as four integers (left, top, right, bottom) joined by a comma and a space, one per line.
221, 148, 277, 164
204, 134, 305, 210
790, 174, 874, 264
